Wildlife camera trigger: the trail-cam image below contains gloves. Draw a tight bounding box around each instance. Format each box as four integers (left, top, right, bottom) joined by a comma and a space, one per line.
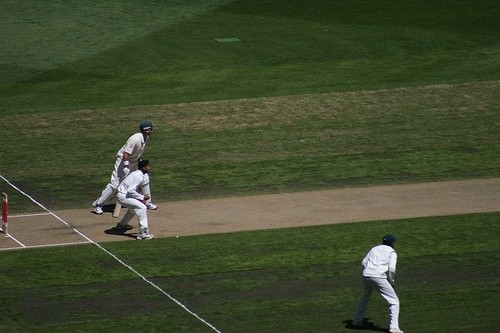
123, 161, 130, 178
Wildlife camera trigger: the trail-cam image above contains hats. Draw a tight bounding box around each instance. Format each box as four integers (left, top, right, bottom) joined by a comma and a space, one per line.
140, 122, 152, 130
383, 235, 393, 242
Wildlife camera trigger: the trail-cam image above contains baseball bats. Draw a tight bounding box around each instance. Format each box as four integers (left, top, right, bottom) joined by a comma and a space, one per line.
112, 200, 122, 218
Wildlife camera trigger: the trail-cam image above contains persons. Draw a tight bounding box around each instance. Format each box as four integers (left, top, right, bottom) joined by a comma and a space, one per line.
92, 121, 158, 214
352, 235, 404, 333
116, 160, 154, 240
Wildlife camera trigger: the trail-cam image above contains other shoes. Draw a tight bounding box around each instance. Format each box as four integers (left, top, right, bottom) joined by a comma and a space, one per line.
117, 223, 132, 230
352, 321, 363, 326
147, 203, 158, 210
389, 328, 404, 333
92, 202, 103, 214
137, 233, 154, 239
